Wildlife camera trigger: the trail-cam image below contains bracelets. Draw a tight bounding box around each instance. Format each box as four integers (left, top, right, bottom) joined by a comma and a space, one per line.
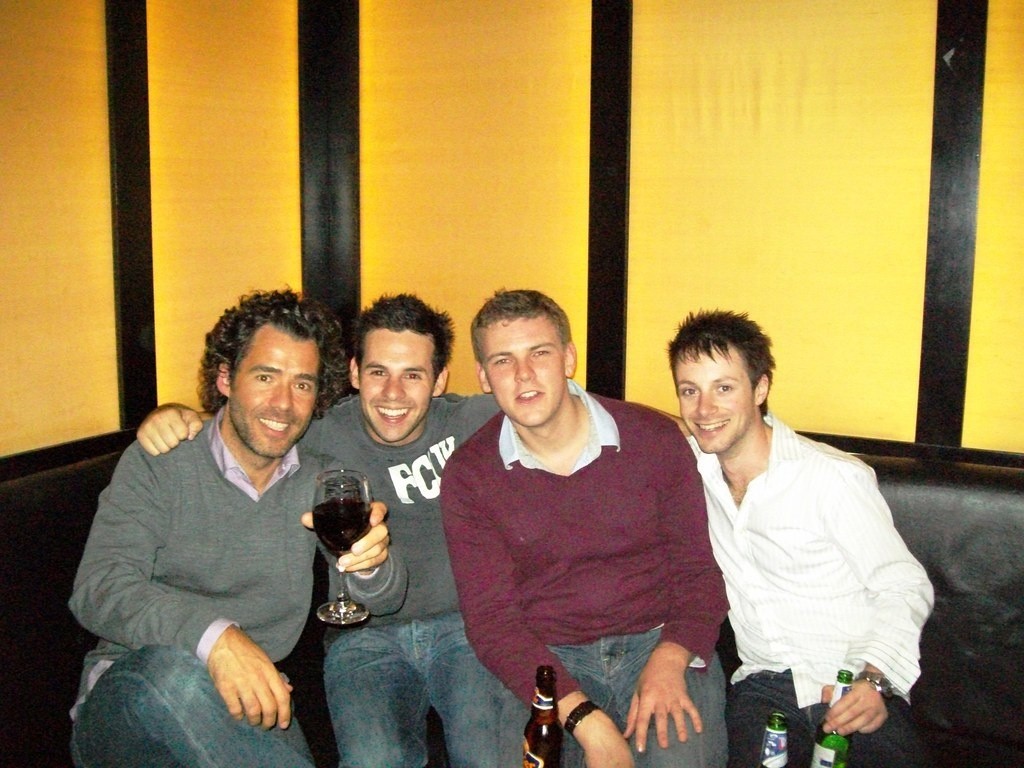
563, 701, 600, 735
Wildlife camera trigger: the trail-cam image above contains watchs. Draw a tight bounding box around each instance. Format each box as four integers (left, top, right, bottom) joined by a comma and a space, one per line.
855, 670, 894, 703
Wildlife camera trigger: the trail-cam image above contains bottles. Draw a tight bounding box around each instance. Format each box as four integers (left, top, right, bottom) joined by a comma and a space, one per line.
810, 670, 853, 768
757, 713, 790, 768
523, 665, 564, 768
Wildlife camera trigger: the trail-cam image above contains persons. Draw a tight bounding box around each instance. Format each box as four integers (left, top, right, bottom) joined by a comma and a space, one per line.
67, 294, 408, 768
666, 312, 934, 768
441, 289, 730, 768
135, 297, 534, 768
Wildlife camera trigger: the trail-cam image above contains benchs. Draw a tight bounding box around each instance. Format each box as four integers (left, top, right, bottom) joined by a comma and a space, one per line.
0, 431, 1024, 768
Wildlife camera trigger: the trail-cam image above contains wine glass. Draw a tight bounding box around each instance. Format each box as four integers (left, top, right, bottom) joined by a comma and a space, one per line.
313, 470, 373, 625
